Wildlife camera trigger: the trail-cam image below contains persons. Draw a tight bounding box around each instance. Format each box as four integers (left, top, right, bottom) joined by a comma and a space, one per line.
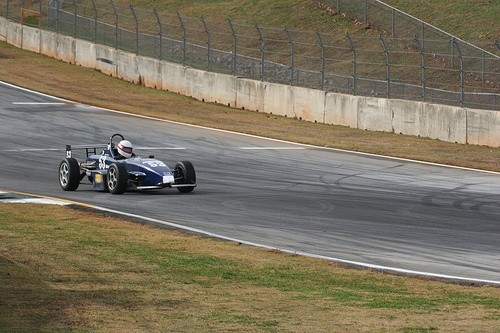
117, 139, 136, 160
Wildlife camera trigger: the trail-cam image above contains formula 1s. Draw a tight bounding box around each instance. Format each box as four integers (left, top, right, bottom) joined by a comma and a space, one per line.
59, 134, 197, 194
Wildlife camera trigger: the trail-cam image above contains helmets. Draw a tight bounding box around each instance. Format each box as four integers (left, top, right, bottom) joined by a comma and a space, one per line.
117, 140, 133, 159
